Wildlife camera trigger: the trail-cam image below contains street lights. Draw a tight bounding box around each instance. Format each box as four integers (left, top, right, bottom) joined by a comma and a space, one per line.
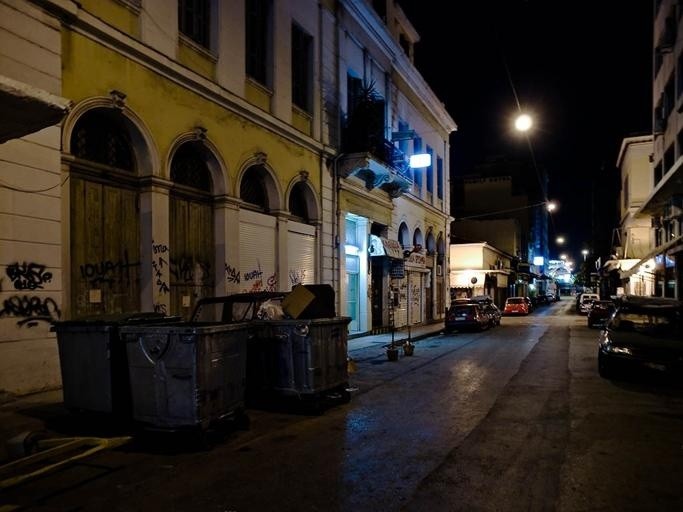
581, 247, 589, 294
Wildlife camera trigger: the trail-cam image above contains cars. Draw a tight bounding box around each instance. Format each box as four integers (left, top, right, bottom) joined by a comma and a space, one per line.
574, 290, 681, 393
504, 293, 562, 315
443, 295, 502, 333
193, 288, 293, 328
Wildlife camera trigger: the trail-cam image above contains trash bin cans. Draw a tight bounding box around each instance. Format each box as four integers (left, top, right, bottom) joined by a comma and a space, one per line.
50, 311, 165, 437
119, 320, 250, 447
246, 317, 352, 415
